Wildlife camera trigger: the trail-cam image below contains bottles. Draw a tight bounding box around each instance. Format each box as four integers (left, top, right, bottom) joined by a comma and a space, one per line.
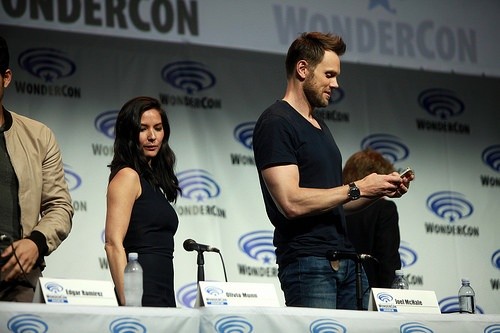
391, 270, 409, 289
458, 279, 476, 313
124, 252, 143, 307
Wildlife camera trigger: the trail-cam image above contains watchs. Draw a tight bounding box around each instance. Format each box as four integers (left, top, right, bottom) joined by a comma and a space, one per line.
348, 182, 360, 200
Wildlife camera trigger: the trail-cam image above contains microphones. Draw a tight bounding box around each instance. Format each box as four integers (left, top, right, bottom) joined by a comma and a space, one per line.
183, 239, 220, 254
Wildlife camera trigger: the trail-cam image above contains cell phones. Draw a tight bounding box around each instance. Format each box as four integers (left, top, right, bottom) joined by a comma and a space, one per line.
398, 169, 413, 184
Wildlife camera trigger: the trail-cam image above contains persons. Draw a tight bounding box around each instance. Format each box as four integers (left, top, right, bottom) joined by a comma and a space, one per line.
104, 96, 182, 308
253, 32, 414, 311
0, 36, 74, 303
342, 149, 402, 289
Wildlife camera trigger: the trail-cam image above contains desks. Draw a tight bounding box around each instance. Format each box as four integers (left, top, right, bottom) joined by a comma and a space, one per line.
0, 301, 500, 333
